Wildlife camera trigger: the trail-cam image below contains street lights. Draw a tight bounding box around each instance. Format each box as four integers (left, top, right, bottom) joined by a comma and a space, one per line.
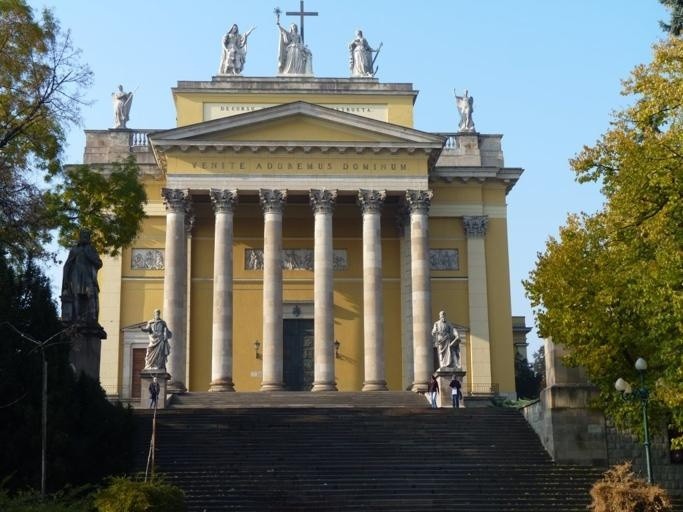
614, 356, 653, 489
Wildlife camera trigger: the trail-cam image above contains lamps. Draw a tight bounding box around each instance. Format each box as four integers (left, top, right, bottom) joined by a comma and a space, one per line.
253, 339, 260, 358
334, 340, 341, 358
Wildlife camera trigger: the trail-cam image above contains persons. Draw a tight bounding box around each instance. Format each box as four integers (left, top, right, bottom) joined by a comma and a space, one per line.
431, 311, 461, 368
141, 309, 172, 370
111, 85, 132, 129
61, 232, 102, 322
429, 375, 439, 408
218, 24, 255, 77
449, 375, 462, 408
277, 24, 312, 75
148, 377, 160, 409
349, 30, 379, 77
453, 87, 475, 132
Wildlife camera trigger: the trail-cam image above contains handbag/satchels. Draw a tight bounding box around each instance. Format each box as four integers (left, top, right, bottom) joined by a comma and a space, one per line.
452, 387, 457, 395
459, 392, 462, 400
